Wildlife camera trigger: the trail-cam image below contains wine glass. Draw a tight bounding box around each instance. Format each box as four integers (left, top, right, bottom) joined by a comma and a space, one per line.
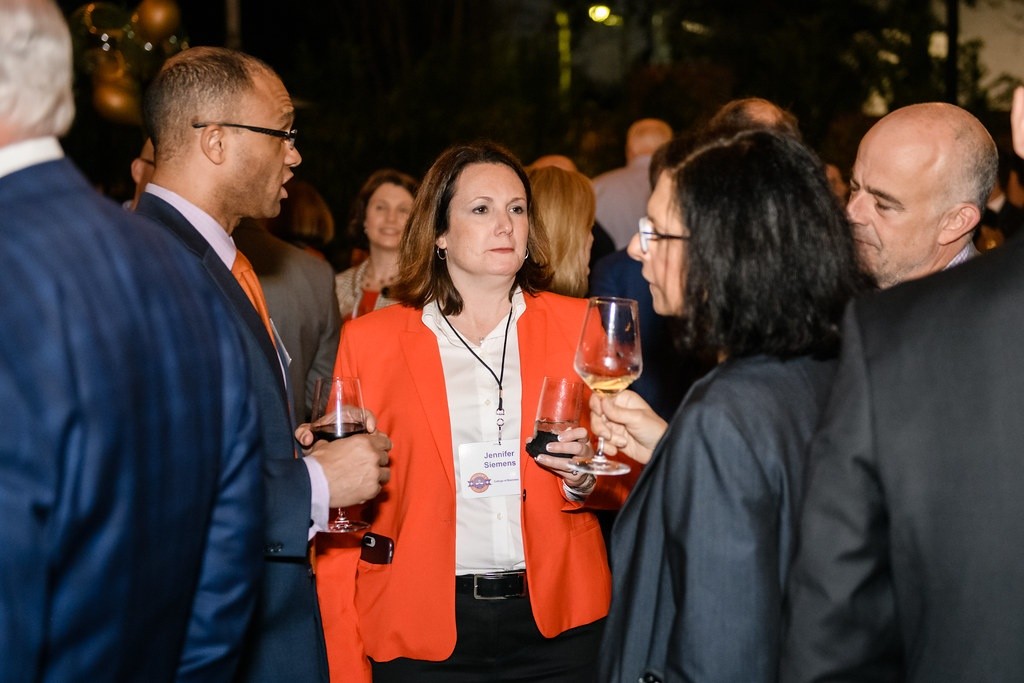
566, 296, 645, 475
309, 375, 372, 533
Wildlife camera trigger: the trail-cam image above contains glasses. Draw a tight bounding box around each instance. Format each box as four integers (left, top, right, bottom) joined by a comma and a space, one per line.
193, 121, 296, 150
638, 215, 692, 253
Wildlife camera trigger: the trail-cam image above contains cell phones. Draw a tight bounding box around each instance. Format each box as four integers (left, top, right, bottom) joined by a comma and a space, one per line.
360, 532, 395, 565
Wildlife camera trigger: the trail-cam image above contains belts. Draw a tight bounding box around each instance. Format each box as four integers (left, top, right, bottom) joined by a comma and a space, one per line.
454, 569, 531, 600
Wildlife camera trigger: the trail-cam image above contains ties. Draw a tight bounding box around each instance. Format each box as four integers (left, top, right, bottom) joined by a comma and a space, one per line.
230, 248, 276, 352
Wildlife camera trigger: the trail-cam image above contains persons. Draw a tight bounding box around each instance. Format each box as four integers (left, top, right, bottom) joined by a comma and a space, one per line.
590, 125, 851, 682
1, 0, 268, 683
310, 143, 643, 683
522, 98, 851, 426
232, 168, 420, 430
773, 85, 1024, 683
119, 48, 390, 683
845, 103, 999, 290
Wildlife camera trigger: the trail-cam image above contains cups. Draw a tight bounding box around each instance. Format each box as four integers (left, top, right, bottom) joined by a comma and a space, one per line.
532, 377, 584, 460
